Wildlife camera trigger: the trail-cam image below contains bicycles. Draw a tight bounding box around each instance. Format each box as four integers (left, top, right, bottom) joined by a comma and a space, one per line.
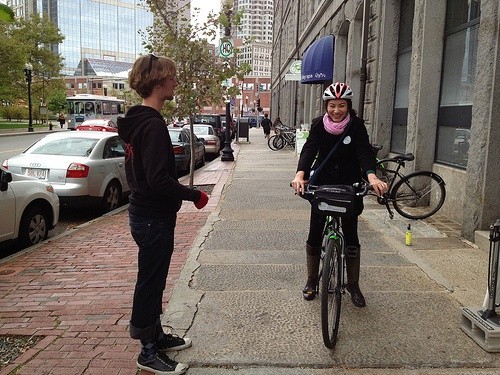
361, 145, 447, 221
267, 124, 299, 151
288, 179, 388, 350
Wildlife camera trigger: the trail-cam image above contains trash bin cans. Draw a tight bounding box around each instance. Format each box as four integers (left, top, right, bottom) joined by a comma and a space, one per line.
236, 122, 249, 142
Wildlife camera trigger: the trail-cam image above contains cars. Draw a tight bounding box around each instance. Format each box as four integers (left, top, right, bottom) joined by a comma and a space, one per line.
0, 164, 59, 247
182, 123, 221, 157
173, 118, 187, 127
1, 130, 131, 215
167, 127, 206, 173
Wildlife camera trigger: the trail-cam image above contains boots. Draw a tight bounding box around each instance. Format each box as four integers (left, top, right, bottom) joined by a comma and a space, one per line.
303, 239, 322, 301
344, 244, 365, 307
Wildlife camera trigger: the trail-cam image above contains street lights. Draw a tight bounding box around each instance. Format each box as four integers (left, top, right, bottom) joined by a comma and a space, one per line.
22, 63, 35, 131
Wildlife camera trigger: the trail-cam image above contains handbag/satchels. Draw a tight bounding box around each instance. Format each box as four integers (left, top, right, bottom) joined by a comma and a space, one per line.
313, 184, 354, 217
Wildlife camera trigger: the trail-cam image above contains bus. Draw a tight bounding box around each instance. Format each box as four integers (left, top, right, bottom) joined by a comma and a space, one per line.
66, 93, 125, 130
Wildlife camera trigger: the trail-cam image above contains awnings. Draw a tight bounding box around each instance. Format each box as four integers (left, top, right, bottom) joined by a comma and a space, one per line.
301, 34, 334, 84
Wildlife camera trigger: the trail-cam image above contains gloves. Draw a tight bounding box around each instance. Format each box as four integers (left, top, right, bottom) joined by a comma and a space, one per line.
194, 191, 208, 209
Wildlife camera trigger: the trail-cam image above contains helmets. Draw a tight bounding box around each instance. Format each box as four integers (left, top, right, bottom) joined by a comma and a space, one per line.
322, 82, 354, 100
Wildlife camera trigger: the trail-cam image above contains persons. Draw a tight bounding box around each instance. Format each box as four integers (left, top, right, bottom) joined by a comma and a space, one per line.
59, 112, 64, 128
117, 54, 209, 375
261, 114, 272, 141
291, 82, 388, 307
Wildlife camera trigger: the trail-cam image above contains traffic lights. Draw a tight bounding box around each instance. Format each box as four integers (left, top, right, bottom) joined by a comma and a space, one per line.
257, 107, 263, 112
256, 99, 260, 106
252, 109, 255, 112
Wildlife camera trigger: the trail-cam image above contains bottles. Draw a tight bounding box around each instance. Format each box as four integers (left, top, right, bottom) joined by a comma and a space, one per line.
406, 224, 412, 246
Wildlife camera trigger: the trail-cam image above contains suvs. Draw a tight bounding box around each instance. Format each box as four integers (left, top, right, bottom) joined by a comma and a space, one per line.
219, 114, 235, 141
194, 114, 225, 147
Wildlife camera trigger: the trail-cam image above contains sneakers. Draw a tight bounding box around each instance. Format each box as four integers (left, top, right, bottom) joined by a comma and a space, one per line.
157, 333, 192, 352
137, 350, 188, 375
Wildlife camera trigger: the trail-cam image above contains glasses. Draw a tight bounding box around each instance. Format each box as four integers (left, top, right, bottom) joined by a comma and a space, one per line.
149, 54, 158, 75
